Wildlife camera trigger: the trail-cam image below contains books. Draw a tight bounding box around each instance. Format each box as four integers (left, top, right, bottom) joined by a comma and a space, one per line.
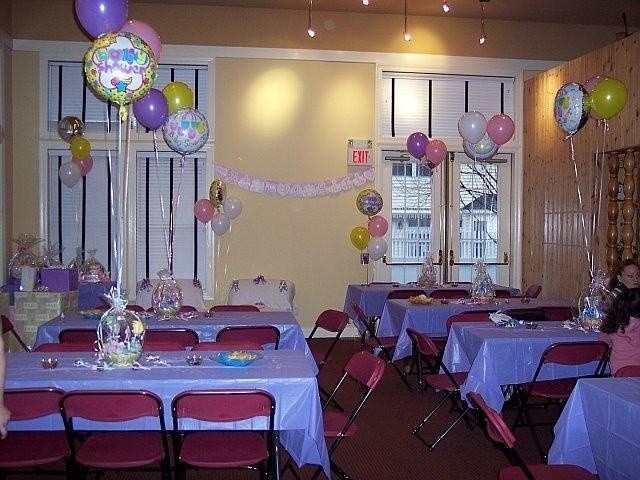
211, 213, 231, 236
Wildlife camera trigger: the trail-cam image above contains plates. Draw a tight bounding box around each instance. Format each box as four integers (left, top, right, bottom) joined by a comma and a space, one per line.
77, 309, 106, 320
209, 351, 264, 367
409, 298, 434, 305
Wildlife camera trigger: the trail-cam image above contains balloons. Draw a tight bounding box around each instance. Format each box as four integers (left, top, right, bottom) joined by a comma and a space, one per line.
582, 75, 612, 125
223, 197, 242, 220
356, 188, 384, 217
552, 81, 591, 135
161, 107, 210, 156
74, 0, 128, 40
425, 139, 447, 166
368, 215, 388, 238
68, 137, 92, 161
365, 238, 388, 263
350, 226, 370, 250
162, 82, 194, 114
59, 162, 81, 187
70, 154, 93, 175
406, 132, 429, 159
118, 18, 162, 67
132, 88, 168, 131
83, 32, 158, 120
193, 199, 215, 223
208, 178, 227, 211
58, 115, 85, 143
486, 113, 515, 145
457, 111, 487, 143
591, 78, 628, 120
462, 132, 499, 161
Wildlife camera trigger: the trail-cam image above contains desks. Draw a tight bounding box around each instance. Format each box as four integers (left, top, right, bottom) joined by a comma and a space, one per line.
548, 377, 640, 480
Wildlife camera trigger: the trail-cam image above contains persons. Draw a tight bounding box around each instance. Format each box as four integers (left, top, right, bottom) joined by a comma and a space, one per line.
599, 258, 640, 313
598, 290, 639, 376
